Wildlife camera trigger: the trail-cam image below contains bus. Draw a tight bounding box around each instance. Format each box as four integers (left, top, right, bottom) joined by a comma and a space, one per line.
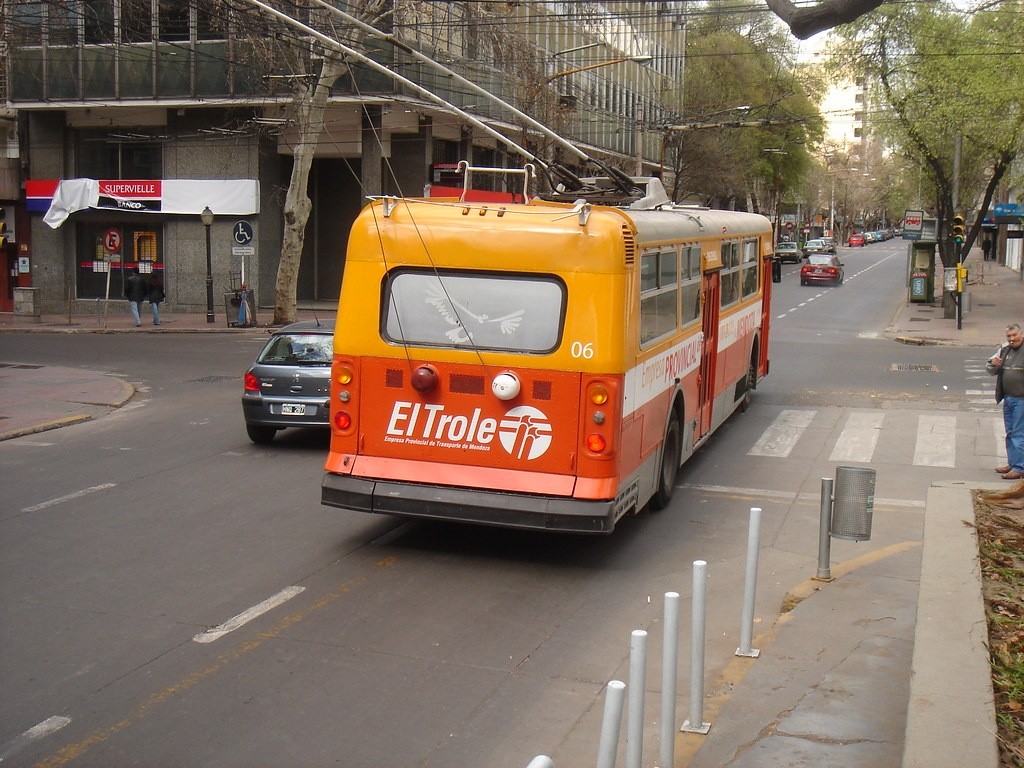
249, 0, 782, 536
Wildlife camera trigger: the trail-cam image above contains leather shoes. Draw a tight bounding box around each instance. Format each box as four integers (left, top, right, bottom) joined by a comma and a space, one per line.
995, 466, 1012, 473
1000, 469, 1024, 479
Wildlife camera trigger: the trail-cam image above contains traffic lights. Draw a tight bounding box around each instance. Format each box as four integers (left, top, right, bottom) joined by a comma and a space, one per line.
952, 213, 966, 244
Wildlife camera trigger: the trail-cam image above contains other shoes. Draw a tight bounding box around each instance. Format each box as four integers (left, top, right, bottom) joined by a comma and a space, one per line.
153, 321, 159, 325
136, 324, 141, 327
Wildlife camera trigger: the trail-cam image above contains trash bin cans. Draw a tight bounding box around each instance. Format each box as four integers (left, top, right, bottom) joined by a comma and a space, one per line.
224, 288, 257, 327
961, 292, 971, 313
828, 465, 876, 542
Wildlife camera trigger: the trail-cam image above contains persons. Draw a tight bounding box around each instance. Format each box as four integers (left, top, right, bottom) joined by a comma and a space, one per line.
146, 272, 164, 326
985, 323, 1023, 479
981, 237, 991, 261
125, 266, 147, 327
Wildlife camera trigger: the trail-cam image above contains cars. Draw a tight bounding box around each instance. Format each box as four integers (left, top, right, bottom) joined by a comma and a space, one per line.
847, 233, 864, 248
242, 318, 338, 446
800, 254, 845, 286
776, 242, 804, 264
804, 239, 829, 256
861, 228, 904, 245
817, 237, 838, 255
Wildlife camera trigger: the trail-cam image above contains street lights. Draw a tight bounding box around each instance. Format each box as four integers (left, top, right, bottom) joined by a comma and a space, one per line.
521, 57, 653, 194
829, 168, 859, 232
659, 105, 751, 188
